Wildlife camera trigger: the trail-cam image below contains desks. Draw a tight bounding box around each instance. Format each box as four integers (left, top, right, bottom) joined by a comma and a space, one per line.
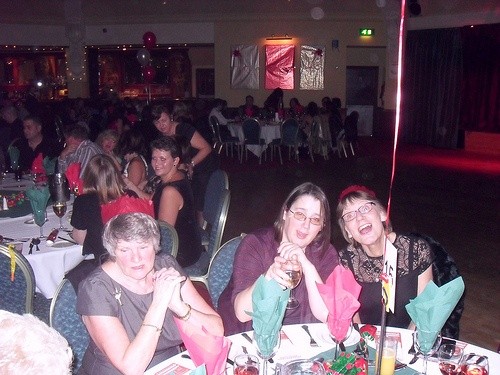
226, 120, 282, 149
0, 172, 95, 298
144, 322, 500, 375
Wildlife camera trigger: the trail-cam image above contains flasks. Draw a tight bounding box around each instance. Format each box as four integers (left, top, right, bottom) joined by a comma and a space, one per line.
54, 173, 70, 201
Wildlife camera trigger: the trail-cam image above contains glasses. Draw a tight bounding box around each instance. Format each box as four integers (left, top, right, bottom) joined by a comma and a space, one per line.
341, 202, 377, 223
288, 207, 326, 226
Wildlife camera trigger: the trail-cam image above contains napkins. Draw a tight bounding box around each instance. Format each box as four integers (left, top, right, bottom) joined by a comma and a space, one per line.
245, 275, 291, 354
172, 315, 232, 375
405, 276, 465, 351
314, 264, 362, 341
25, 185, 50, 222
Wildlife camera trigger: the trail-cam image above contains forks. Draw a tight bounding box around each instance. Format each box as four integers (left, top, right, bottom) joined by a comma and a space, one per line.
302, 325, 321, 348
28, 242, 33, 255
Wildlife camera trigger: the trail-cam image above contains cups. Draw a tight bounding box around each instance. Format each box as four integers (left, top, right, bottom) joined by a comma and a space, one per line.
233, 354, 260, 375
375, 332, 400, 375
437, 344, 464, 375
460, 354, 490, 375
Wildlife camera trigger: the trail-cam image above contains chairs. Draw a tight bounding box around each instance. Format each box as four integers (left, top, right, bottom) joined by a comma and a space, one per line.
0, 242, 94, 375
4, 139, 23, 165
154, 169, 244, 305
210, 111, 361, 165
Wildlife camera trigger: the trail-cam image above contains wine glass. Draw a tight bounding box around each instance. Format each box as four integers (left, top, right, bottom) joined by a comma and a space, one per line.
413, 330, 442, 375
32, 209, 48, 241
326, 312, 353, 369
52, 200, 68, 231
281, 260, 302, 310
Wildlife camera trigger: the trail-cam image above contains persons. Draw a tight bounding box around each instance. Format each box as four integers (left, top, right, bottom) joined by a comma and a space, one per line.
208, 94, 260, 153
148, 103, 212, 232
334, 184, 444, 333
72, 211, 224, 375
216, 181, 343, 338
0, 91, 202, 285
263, 87, 349, 139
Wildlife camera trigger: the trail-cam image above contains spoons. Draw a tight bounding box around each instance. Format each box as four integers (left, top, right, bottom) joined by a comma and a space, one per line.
33, 239, 40, 251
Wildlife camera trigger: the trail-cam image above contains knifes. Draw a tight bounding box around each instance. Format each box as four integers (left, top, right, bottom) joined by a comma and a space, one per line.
241, 333, 274, 363
58, 236, 79, 245
226, 358, 238, 366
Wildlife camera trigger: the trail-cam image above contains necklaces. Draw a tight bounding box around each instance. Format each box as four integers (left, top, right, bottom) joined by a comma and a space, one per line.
29, 140, 37, 147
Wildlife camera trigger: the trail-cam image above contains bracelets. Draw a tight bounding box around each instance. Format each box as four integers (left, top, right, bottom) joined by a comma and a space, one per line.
142, 322, 163, 334
169, 302, 191, 321
191, 159, 195, 167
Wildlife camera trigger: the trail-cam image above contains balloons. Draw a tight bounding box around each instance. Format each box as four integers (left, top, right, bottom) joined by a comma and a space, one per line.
137, 32, 156, 81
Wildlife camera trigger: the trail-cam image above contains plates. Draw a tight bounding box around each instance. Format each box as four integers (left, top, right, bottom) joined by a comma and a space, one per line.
323, 326, 361, 347
